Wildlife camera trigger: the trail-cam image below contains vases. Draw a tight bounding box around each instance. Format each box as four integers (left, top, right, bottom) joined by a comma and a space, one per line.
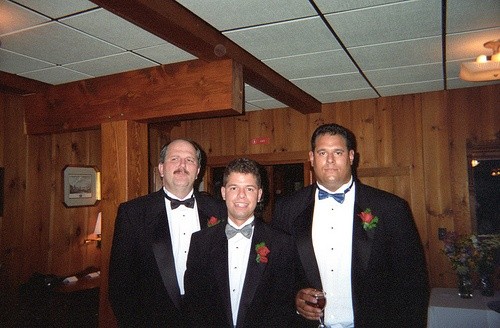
456, 272, 494, 298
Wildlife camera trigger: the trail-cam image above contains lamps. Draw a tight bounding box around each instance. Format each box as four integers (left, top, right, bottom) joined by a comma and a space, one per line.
460, 39, 500, 82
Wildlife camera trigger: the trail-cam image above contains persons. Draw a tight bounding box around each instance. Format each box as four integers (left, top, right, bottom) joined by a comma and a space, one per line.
271, 125, 429, 328
184, 157, 298, 328
107, 139, 222, 328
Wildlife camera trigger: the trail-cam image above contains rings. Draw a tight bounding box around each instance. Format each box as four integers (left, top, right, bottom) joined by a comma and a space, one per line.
297, 311, 299, 315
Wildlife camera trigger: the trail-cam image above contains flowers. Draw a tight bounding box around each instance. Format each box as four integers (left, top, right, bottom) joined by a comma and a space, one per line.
441, 231, 500, 273
207, 216, 220, 228
256, 242, 270, 263
359, 207, 379, 230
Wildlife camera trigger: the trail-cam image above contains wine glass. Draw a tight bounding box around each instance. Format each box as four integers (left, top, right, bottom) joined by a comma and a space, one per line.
305, 290, 331, 328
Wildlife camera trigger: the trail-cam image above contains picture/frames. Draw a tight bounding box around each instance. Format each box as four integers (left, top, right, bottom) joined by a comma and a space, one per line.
61, 165, 101, 207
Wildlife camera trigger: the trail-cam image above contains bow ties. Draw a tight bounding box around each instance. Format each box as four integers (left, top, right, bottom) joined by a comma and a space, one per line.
225, 221, 254, 239
164, 192, 195, 210
316, 180, 354, 204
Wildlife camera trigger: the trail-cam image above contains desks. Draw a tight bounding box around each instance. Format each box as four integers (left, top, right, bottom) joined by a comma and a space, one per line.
427, 288, 500, 328
